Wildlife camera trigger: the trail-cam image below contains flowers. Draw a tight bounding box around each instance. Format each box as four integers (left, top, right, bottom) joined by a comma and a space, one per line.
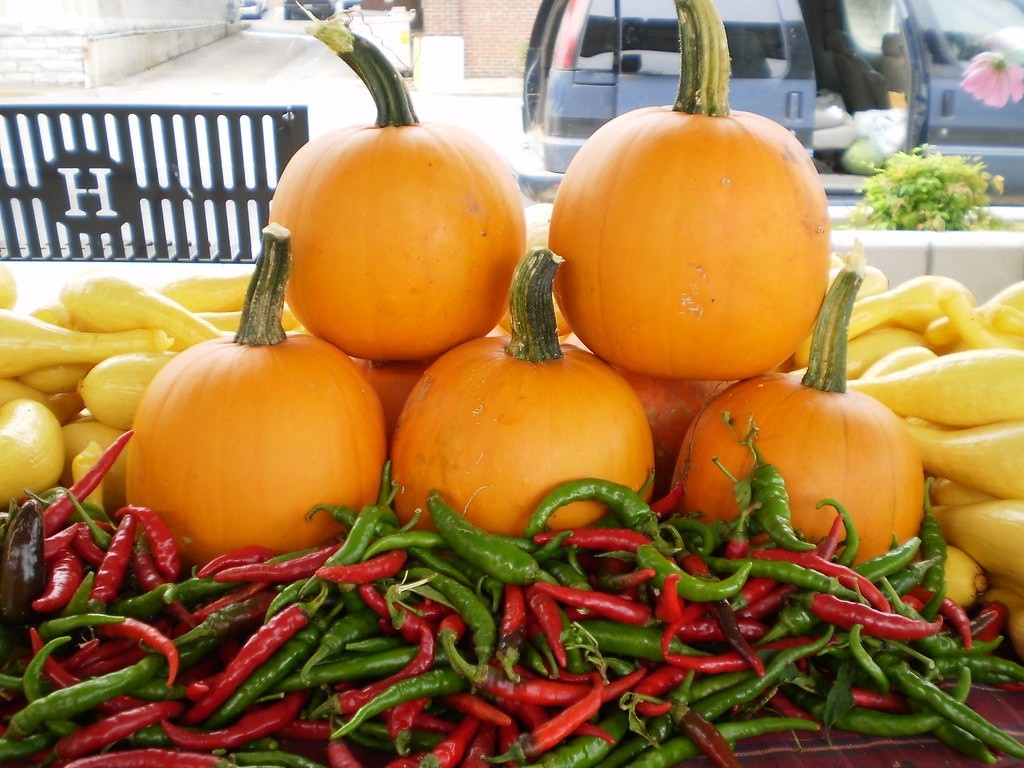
962, 50, 1024, 107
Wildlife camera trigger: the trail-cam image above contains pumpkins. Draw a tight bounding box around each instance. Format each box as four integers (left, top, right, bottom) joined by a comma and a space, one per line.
121, 1, 922, 563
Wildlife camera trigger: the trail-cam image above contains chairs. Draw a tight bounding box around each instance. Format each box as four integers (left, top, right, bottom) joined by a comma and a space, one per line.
877, 35, 912, 101
812, 86, 857, 152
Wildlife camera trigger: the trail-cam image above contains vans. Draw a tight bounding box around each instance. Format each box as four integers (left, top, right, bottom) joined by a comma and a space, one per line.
515, 0, 1023, 239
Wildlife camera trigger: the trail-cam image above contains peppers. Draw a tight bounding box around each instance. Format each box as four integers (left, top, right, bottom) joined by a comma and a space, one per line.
0, 431, 1024, 766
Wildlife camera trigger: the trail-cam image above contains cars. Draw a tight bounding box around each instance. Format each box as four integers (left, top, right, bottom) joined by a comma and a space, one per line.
282, 0, 339, 22
239, 0, 269, 21
